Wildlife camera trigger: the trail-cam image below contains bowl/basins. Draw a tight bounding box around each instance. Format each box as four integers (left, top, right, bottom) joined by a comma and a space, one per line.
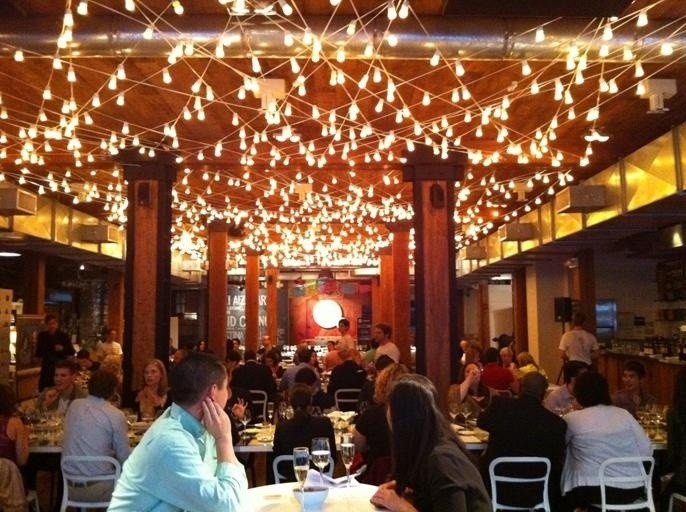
292, 485, 329, 511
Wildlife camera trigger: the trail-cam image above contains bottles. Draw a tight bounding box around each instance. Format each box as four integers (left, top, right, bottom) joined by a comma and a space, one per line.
642, 334, 686, 357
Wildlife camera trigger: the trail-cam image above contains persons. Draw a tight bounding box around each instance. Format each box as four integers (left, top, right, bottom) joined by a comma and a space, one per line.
559, 312, 600, 384
643, 367, 686, 512
1, 309, 337, 512
328, 319, 653, 512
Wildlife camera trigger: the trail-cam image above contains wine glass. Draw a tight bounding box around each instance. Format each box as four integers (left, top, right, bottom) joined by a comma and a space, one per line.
461, 401, 473, 426
447, 401, 461, 425
339, 443, 356, 486
292, 445, 310, 489
281, 344, 321, 356
635, 401, 668, 441
266, 401, 274, 426
237, 408, 251, 441
119, 408, 138, 436
21, 405, 66, 442
310, 437, 332, 484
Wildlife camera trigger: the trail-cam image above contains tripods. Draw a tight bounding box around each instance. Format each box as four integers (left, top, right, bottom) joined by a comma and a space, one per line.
556, 323, 566, 385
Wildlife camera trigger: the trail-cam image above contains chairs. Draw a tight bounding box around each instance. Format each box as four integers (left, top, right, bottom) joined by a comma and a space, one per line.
273, 453, 336, 484
487, 455, 553, 512
57, 453, 122, 512
23, 340, 673, 455
594, 454, 660, 512
0, 458, 41, 512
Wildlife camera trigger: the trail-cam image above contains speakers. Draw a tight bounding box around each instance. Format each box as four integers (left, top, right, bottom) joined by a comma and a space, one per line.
554, 297, 572, 322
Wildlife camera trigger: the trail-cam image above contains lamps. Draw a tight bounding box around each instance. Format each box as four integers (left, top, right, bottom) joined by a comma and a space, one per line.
236, 283, 244, 292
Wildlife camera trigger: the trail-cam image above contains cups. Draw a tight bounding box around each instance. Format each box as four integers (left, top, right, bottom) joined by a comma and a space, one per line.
285, 409, 294, 420
476, 368, 484, 376
141, 417, 153, 428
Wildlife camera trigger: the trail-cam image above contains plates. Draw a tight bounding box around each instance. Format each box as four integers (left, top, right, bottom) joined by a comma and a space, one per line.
456, 428, 476, 436
254, 432, 274, 442
